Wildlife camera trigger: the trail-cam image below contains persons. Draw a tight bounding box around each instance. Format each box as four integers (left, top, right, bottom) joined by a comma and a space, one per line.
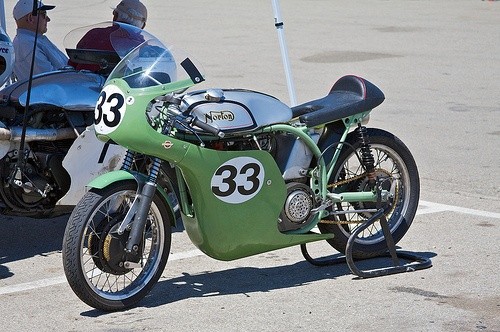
68, 0, 148, 72
12, 0, 70, 81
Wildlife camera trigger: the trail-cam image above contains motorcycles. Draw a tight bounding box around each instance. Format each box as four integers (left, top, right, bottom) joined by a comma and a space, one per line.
62, 21, 420, 312
0, 69, 106, 217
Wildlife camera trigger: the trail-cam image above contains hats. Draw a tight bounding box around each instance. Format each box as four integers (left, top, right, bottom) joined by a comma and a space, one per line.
12, 0, 56, 20
110, 0, 148, 21
